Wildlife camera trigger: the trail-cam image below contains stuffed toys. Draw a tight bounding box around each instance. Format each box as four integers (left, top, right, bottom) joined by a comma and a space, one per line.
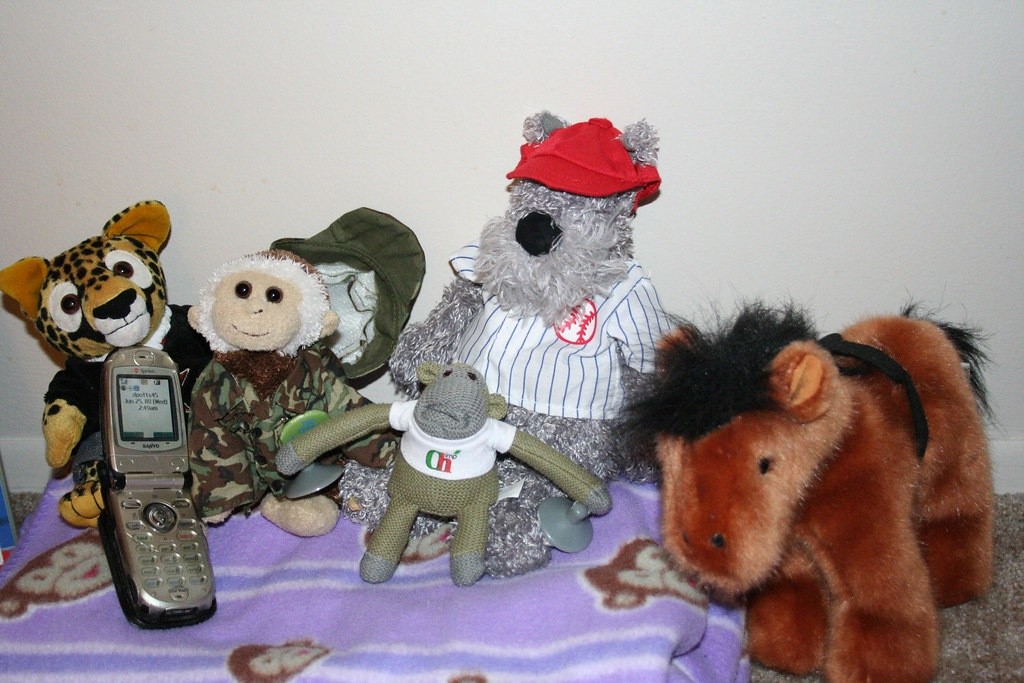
0, 109, 995, 683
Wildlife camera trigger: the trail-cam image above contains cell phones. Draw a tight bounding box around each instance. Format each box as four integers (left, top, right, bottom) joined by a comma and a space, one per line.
96, 345, 215, 625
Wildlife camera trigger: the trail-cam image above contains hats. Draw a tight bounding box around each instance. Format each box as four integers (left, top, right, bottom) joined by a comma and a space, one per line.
271, 208, 427, 379
505, 118, 661, 216
